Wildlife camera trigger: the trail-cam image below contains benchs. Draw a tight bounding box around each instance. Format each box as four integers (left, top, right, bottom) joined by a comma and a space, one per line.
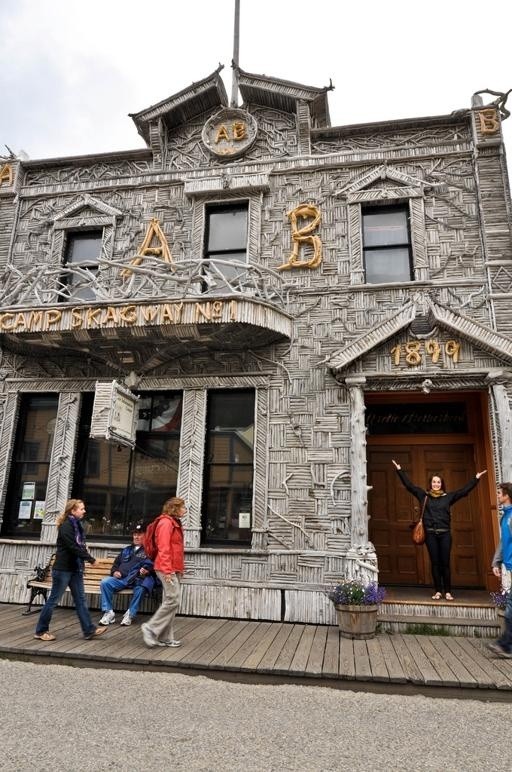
24, 550, 161, 625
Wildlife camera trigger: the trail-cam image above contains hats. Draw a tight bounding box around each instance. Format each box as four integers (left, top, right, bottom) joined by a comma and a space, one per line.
129, 522, 148, 534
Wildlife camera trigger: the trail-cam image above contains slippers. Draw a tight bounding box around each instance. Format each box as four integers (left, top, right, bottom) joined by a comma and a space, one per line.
431, 591, 442, 601
446, 592, 455, 601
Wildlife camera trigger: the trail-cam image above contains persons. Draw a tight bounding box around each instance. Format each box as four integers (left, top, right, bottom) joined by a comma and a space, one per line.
392, 459, 487, 600
486, 482, 512, 659
140, 498, 187, 647
99, 523, 156, 626
34, 500, 108, 641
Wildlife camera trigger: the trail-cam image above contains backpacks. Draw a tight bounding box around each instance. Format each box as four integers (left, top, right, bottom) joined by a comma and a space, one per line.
142, 514, 175, 560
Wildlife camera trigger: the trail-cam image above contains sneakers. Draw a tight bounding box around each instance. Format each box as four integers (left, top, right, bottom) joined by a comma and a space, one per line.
156, 638, 182, 646
33, 631, 56, 641
140, 622, 156, 648
98, 609, 117, 626
84, 627, 107, 641
486, 638, 512, 659
121, 609, 133, 627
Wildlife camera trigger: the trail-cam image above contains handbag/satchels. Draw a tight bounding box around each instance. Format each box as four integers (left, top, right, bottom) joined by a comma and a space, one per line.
411, 518, 428, 546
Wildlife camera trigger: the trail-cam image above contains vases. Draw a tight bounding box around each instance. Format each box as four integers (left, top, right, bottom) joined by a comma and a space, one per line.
337, 600, 381, 642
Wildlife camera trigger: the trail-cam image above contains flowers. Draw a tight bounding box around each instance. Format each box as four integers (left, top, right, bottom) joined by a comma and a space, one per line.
321, 573, 387, 607
